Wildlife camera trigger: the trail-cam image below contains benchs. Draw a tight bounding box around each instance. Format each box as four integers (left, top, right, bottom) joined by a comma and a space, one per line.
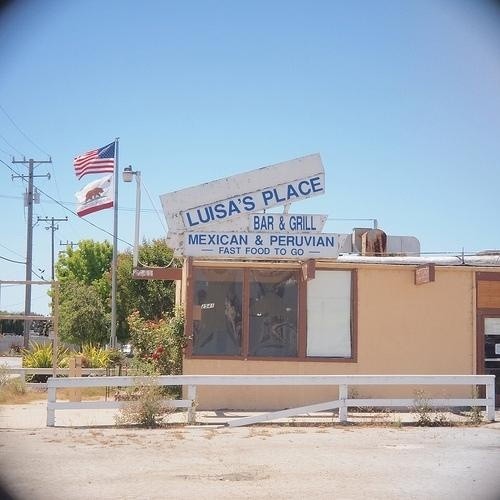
74, 141, 115, 180
76, 173, 113, 218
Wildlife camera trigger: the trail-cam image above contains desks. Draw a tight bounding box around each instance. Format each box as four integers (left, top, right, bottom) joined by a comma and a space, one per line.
123, 165, 140, 272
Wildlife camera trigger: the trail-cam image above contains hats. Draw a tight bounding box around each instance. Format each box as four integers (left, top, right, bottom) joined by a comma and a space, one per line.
119, 344, 133, 357
